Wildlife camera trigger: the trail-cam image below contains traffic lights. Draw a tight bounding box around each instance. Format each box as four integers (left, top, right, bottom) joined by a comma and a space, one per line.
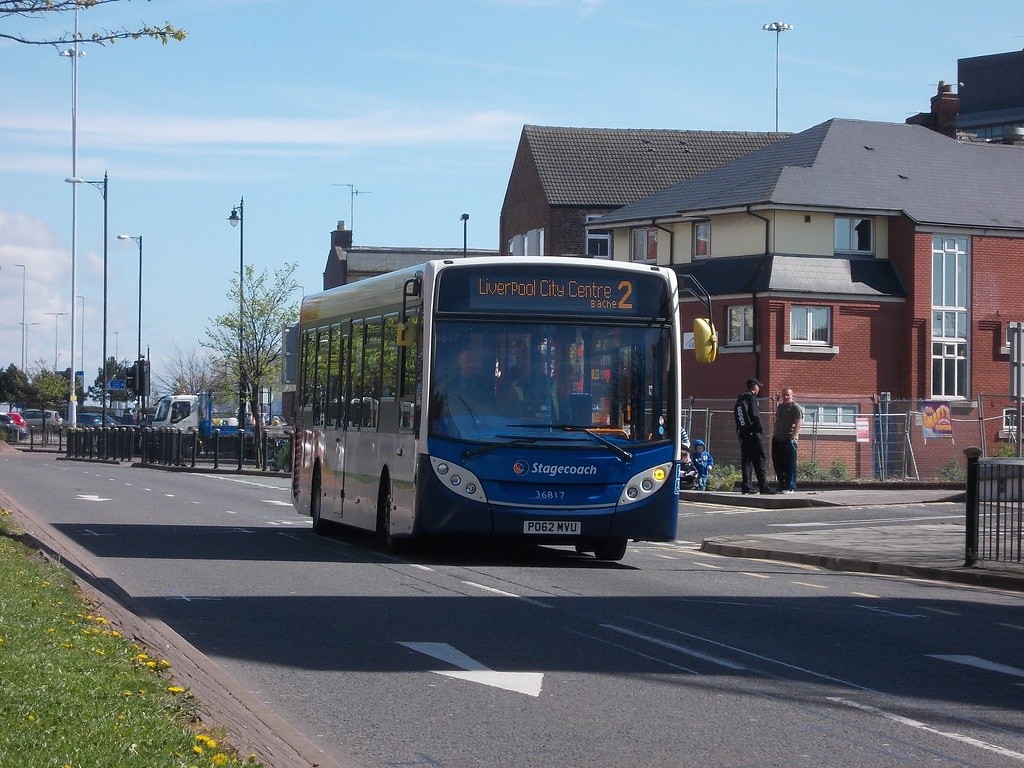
125, 364, 139, 393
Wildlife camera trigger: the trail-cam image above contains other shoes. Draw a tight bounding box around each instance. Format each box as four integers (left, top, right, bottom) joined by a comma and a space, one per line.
741, 488, 760, 496
781, 489, 794, 494
759, 486, 780, 494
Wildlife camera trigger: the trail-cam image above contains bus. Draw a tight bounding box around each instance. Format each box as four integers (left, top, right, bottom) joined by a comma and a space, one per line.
288, 256, 719, 564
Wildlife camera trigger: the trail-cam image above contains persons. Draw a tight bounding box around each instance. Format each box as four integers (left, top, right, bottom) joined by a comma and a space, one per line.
734, 377, 778, 494
432, 347, 502, 420
680, 426, 714, 491
771, 388, 804, 494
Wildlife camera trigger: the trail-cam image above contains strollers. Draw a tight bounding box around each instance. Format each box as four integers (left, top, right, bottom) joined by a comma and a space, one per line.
681, 447, 700, 490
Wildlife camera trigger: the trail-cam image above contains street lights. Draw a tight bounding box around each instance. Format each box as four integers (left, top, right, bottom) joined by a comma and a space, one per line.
17, 320, 40, 372
762, 21, 792, 133
225, 195, 249, 429
116, 234, 142, 427
64, 169, 107, 429
77, 295, 85, 372
112, 331, 119, 364
14, 264, 26, 376
460, 213, 469, 258
46, 311, 69, 375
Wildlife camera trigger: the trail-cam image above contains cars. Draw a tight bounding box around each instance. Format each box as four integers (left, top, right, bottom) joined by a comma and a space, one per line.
0, 412, 29, 440
76, 412, 122, 429
20, 409, 64, 427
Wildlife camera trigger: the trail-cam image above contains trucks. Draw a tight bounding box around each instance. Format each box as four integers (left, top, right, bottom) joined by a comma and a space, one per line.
149, 389, 295, 437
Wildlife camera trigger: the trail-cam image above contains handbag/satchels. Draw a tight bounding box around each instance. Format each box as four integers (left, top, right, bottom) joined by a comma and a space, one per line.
747, 433, 766, 456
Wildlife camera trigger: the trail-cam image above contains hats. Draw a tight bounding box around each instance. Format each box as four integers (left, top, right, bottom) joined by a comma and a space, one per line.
746, 378, 764, 387
